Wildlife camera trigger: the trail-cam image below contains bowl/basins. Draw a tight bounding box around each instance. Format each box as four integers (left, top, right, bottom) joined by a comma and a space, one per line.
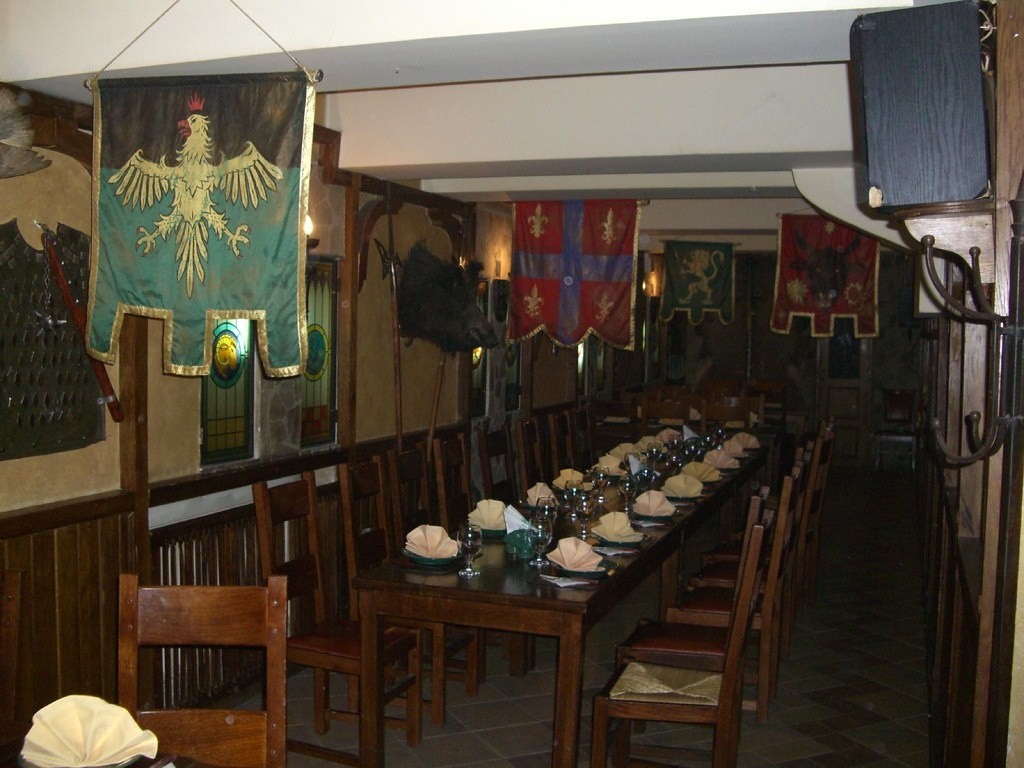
503, 529, 536, 558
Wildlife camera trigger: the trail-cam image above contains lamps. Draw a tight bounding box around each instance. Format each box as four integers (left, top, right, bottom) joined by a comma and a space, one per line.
305, 214, 320, 256
491, 279, 510, 323
650, 295, 662, 324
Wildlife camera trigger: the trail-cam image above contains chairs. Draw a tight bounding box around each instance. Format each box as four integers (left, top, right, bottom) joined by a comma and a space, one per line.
337, 453, 477, 726
252, 470, 422, 757
477, 377, 835, 768
117, 573, 288, 768
387, 440, 530, 685
872, 388, 917, 473
432, 432, 538, 665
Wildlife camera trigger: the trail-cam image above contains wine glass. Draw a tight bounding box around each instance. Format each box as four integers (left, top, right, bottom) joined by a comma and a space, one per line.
528, 518, 556, 567
564, 480, 581, 520
536, 497, 558, 540
620, 429, 731, 523
576, 494, 593, 541
592, 466, 611, 503
457, 526, 483, 577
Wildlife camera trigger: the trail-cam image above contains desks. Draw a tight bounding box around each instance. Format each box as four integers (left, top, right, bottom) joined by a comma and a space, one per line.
352, 431, 786, 768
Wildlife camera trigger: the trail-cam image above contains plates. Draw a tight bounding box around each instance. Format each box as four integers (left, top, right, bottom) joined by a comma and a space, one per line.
481, 528, 506, 541
635, 448, 759, 525
403, 549, 463, 574
17, 755, 143, 768
590, 532, 649, 547
549, 558, 618, 580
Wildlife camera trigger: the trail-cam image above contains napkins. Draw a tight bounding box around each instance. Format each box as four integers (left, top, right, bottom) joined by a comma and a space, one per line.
20, 695, 159, 768
546, 536, 603, 571
552, 469, 593, 491
680, 462, 722, 482
684, 425, 701, 441
609, 443, 647, 461
632, 489, 677, 516
629, 455, 661, 477
635, 436, 668, 454
704, 449, 740, 469
405, 482, 560, 559
590, 512, 644, 543
591, 453, 628, 475
723, 441, 752, 457
656, 428, 682, 443
660, 474, 704, 498
731, 432, 761, 448
